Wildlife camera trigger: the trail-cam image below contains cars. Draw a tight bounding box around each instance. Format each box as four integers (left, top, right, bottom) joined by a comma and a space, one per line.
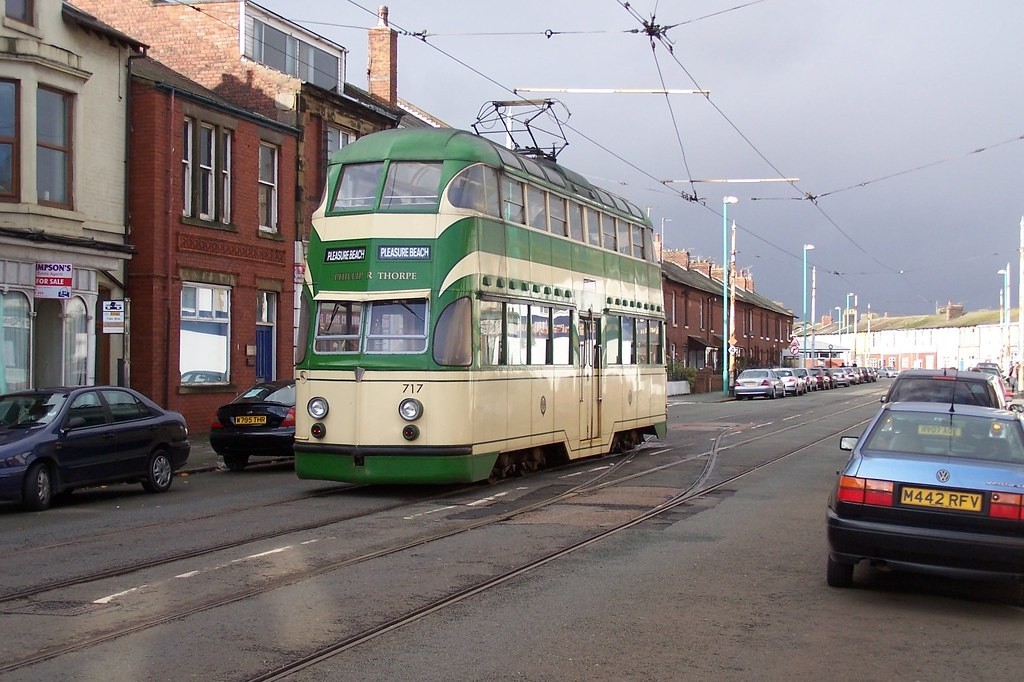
792, 366, 879, 394
880, 368, 1024, 455
208, 379, 295, 473
969, 362, 1006, 380
734, 369, 786, 399
771, 368, 803, 396
824, 346, 1024, 606
0, 385, 192, 512
877, 366, 901, 378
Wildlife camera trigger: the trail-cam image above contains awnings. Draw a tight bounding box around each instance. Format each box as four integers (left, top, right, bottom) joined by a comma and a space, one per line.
714, 334, 744, 348
689, 335, 718, 348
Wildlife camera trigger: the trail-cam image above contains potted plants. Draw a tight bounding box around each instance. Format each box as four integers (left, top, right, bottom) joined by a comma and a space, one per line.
667, 358, 697, 396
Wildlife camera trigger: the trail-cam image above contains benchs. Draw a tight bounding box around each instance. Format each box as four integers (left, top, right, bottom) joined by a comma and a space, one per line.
891, 432, 1013, 463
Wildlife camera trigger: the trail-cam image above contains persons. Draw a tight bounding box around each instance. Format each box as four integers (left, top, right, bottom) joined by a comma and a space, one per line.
1009, 362, 1020, 393
841, 363, 845, 367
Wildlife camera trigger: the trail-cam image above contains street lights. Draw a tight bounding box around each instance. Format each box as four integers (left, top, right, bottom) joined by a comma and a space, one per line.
997, 269, 1007, 327
803, 243, 815, 368
834, 306, 841, 345
847, 292, 854, 346
722, 195, 739, 398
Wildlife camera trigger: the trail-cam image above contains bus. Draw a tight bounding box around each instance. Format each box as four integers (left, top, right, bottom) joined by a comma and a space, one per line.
294, 126, 670, 488
